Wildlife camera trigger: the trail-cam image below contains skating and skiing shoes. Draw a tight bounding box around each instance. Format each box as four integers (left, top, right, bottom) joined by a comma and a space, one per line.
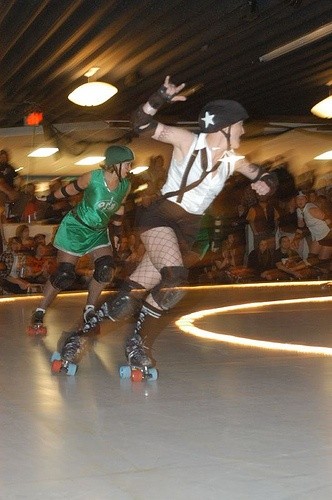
80, 305, 99, 333
119, 340, 158, 383
25, 308, 46, 335
51, 334, 85, 377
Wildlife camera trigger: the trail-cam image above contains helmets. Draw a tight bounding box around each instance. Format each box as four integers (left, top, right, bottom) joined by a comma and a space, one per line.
198, 100, 250, 133
104, 145, 134, 167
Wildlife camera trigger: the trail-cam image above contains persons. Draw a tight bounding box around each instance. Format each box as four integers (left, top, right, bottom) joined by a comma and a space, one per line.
62, 75, 277, 365
0, 150, 332, 295
31, 145, 134, 328
